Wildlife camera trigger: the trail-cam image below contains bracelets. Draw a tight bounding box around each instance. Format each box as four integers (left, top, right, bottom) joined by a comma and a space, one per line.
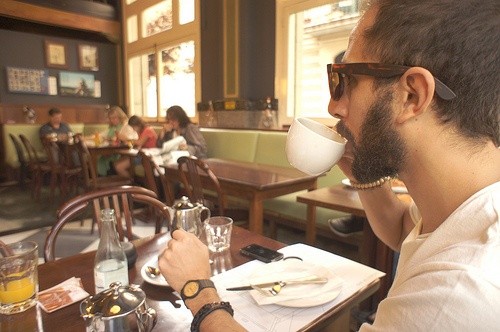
190, 301, 235, 332
350, 175, 388, 189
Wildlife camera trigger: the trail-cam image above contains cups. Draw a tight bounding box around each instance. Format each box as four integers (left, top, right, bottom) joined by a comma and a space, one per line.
0, 241, 40, 314
284, 116, 349, 177
202, 215, 233, 252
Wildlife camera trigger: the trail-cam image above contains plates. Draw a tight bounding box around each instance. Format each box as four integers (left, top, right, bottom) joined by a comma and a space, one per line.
140, 249, 177, 287
249, 263, 340, 307
342, 178, 355, 188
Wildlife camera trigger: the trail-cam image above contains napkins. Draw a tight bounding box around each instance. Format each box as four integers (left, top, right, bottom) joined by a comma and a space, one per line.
248, 262, 343, 306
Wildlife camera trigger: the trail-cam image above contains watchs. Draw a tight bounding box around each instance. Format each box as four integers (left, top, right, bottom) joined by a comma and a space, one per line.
180, 278, 217, 308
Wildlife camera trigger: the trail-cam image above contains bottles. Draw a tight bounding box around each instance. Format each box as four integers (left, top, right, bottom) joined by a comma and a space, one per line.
93, 209, 130, 297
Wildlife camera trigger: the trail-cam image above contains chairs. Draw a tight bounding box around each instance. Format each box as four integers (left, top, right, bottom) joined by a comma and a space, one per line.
8, 133, 249, 262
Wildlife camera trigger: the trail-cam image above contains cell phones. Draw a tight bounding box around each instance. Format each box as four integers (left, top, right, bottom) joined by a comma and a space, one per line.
241, 243, 284, 263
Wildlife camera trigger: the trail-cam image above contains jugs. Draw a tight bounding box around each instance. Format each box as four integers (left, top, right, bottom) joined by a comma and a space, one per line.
79, 280, 158, 332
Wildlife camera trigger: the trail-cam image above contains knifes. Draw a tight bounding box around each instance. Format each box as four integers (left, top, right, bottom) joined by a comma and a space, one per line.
226, 276, 331, 294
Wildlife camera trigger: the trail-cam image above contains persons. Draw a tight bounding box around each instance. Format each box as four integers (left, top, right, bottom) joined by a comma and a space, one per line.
157, 0, 500, 332
40, 105, 207, 202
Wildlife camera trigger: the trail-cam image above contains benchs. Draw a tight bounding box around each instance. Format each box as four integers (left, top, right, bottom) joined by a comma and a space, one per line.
83, 124, 365, 247
1, 121, 84, 189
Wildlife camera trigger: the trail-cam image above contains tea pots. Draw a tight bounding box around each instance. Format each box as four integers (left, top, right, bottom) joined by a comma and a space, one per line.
162, 194, 211, 240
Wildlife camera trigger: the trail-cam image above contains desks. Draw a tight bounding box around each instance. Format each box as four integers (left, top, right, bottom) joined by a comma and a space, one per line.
295, 185, 413, 332
0, 222, 381, 332
50, 137, 327, 246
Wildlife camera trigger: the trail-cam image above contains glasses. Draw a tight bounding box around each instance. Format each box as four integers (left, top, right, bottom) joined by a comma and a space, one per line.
326, 63, 456, 102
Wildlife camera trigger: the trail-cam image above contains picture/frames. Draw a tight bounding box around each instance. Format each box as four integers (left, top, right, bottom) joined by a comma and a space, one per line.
44, 38, 69, 70
76, 42, 99, 71
